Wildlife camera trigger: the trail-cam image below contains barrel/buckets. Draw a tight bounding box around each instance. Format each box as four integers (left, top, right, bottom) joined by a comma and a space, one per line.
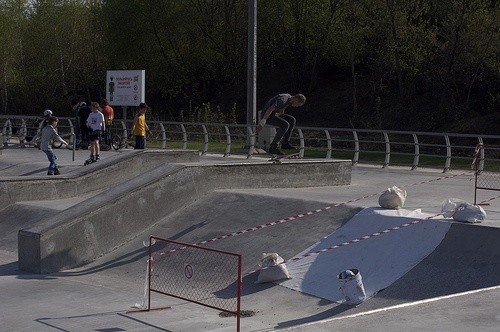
337, 269, 366, 304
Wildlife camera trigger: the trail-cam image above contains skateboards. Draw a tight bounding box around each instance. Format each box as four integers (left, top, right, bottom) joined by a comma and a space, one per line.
268, 149, 301, 165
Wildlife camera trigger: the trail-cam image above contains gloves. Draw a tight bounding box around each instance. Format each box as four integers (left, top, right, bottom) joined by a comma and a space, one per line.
260, 118, 266, 127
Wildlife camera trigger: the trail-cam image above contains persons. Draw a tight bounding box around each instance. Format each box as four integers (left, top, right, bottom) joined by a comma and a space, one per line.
73, 99, 102, 150
85, 101, 105, 161
40, 116, 69, 175
1, 117, 38, 149
38, 109, 58, 144
100, 98, 114, 145
256, 93, 306, 155
130, 102, 154, 149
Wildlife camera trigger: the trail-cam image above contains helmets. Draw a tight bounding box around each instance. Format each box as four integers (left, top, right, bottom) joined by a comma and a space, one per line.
44, 110, 53, 116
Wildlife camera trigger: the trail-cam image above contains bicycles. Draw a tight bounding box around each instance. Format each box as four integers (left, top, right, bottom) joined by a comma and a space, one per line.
100, 125, 121, 151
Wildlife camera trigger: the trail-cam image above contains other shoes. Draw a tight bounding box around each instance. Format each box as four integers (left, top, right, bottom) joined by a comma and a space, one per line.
269, 147, 285, 155
281, 143, 297, 150
90, 155, 94, 160
96, 154, 100, 160
